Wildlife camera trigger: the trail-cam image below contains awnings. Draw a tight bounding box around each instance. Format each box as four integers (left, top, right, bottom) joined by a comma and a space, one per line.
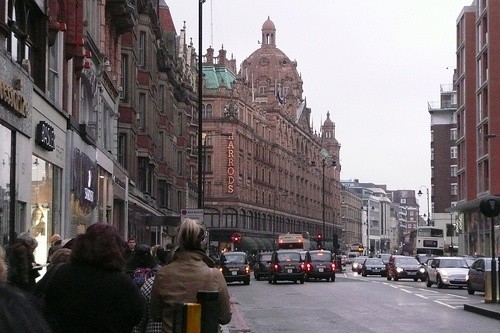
310, 241, 348, 250
240, 237, 279, 251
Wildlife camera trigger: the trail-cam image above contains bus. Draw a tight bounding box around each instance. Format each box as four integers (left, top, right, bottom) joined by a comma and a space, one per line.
409, 227, 445, 257
278, 230, 310, 251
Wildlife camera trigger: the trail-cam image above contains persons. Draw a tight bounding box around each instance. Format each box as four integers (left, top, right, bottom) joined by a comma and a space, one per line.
31, 208, 45, 237
43, 222, 147, 333
0, 232, 227, 333
149, 218, 232, 333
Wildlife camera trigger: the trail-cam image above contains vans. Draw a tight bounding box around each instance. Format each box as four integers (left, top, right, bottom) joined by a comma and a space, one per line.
444, 245, 458, 257
348, 252, 361, 263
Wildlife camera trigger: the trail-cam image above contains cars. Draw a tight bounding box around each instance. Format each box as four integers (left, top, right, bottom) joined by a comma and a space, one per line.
220, 252, 251, 285
254, 249, 346, 284
353, 253, 499, 294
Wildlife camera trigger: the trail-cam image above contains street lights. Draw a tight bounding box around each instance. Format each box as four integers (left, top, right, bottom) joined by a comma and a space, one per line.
418, 185, 430, 225
317, 159, 337, 248
361, 205, 374, 256
